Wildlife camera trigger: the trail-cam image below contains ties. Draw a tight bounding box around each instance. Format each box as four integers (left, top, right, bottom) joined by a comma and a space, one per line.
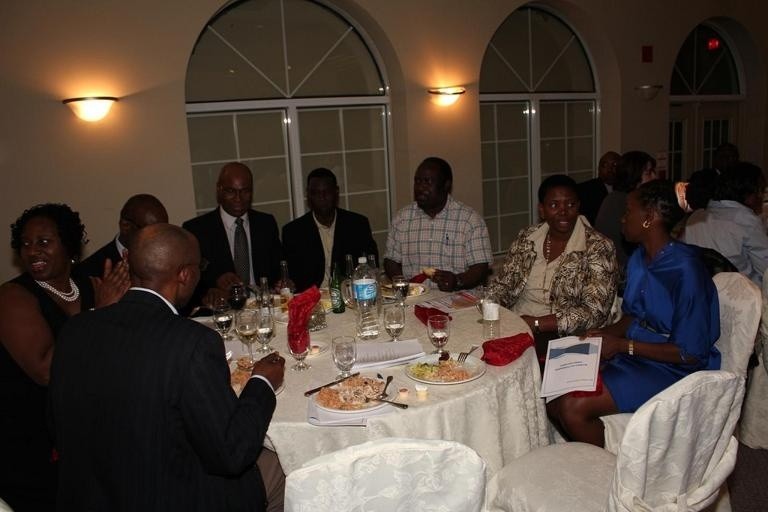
235, 214, 249, 289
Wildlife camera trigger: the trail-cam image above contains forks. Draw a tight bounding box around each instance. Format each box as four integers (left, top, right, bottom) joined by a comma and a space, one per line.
406, 353, 485, 384
457, 346, 477, 362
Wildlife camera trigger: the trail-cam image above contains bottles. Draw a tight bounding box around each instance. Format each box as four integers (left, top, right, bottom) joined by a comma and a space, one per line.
483, 286, 501, 340
351, 256, 381, 341
278, 263, 298, 302
258, 275, 272, 311
329, 261, 346, 315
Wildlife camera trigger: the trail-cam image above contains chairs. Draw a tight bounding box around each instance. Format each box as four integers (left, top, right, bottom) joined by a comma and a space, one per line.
282, 440, 485, 512
737, 267, 768, 449
711, 273, 764, 371
494, 371, 746, 512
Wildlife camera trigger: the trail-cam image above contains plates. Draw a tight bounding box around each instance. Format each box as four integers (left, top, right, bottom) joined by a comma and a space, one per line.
245, 295, 280, 311
381, 282, 429, 299
314, 378, 396, 414
231, 359, 285, 396
193, 316, 216, 329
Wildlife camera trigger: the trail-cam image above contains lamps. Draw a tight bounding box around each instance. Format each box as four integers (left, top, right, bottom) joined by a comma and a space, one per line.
425, 87, 468, 110
65, 94, 120, 121
632, 84, 663, 102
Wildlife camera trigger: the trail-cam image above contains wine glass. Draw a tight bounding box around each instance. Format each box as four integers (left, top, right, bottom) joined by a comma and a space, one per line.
427, 315, 450, 353
287, 327, 312, 373
383, 305, 405, 342
236, 310, 256, 358
391, 275, 408, 303
213, 306, 233, 341
475, 286, 487, 323
331, 336, 356, 381
255, 313, 274, 354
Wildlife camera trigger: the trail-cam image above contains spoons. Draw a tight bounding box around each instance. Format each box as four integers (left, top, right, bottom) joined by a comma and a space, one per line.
376, 376, 394, 401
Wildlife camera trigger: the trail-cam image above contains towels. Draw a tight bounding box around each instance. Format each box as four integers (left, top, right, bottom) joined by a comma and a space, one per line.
482, 332, 538, 368
408, 274, 428, 283
287, 284, 321, 354
570, 371, 603, 398
414, 305, 451, 330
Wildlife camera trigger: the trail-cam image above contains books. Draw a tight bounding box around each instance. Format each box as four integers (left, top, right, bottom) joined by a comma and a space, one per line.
540, 335, 605, 404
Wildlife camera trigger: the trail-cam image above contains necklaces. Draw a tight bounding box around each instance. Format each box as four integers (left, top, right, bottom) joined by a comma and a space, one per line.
542, 232, 565, 304
35, 278, 79, 302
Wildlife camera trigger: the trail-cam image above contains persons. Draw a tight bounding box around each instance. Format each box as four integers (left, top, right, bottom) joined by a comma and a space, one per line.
0, 204, 130, 512
575, 152, 620, 227
679, 161, 768, 287
281, 168, 379, 295
476, 175, 618, 339
182, 163, 295, 298
595, 151, 657, 297
47, 223, 285, 512
383, 158, 493, 292
82, 193, 230, 312
543, 178, 722, 449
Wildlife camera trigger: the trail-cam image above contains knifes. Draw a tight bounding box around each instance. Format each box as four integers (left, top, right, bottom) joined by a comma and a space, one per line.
305, 372, 361, 397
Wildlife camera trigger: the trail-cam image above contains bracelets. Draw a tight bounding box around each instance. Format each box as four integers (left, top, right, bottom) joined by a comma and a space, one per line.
628, 339, 633, 357
535, 317, 540, 335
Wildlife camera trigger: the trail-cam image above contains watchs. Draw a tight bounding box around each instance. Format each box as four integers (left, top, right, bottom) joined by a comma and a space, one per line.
455, 275, 463, 291
